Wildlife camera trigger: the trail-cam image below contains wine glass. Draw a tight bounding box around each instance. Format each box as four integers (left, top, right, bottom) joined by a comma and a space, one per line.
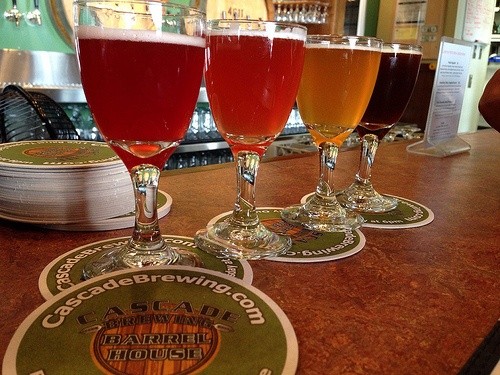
73, 1, 208, 291
336, 37, 422, 216
204, 16, 307, 260
280, 33, 383, 229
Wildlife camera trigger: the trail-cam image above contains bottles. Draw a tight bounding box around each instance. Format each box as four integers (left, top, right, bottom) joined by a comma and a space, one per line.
278, 1, 330, 24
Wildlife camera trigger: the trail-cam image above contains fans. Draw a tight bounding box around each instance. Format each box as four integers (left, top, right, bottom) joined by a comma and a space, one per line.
0, 84, 80, 141
